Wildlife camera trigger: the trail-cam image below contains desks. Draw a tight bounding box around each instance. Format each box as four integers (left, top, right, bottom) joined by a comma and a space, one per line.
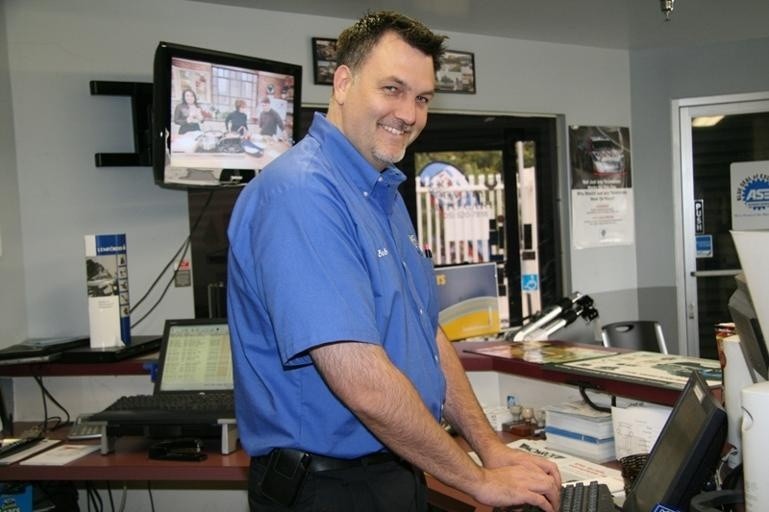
425, 339, 722, 511
1, 436, 251, 512
0, 335, 159, 437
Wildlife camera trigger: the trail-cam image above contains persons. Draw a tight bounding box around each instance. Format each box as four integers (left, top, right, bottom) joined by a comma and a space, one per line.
226, 100, 249, 134
257, 98, 288, 139
174, 89, 205, 136
226, 12, 561, 512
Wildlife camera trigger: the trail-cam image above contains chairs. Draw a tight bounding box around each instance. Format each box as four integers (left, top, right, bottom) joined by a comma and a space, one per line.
579, 320, 668, 413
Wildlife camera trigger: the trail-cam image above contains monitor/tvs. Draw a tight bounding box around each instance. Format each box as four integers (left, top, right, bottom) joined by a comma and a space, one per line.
622, 369, 728, 512
154, 318, 235, 395
153, 42, 302, 190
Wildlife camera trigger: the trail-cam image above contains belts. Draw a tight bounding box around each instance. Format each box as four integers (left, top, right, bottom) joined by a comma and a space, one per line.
261, 448, 403, 478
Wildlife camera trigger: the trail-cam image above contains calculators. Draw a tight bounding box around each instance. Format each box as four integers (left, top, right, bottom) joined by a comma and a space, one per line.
67, 413, 109, 440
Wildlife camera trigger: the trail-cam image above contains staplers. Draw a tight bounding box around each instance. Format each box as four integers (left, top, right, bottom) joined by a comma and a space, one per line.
148, 438, 207, 461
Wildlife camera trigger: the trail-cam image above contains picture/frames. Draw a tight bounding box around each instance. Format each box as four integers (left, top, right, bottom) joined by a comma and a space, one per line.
311, 36, 475, 94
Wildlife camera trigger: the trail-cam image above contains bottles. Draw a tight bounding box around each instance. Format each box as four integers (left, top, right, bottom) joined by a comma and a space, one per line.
715, 322, 738, 386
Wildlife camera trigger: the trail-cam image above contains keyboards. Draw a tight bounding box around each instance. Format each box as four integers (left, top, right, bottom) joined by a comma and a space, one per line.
87, 394, 235, 421
523, 480, 614, 512
